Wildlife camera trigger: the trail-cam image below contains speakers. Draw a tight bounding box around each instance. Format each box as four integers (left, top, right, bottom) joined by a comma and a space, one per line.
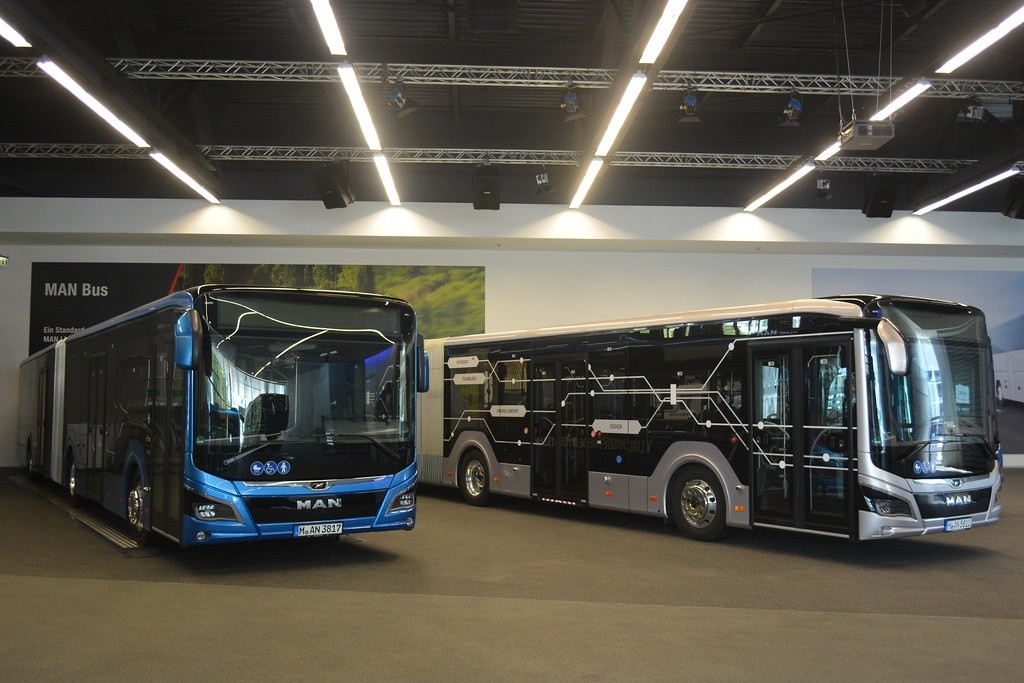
861, 174, 898, 218
472, 164, 501, 211
311, 162, 358, 210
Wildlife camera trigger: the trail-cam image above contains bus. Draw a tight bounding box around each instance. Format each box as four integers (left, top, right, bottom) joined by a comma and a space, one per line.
64, 358, 168, 468
18, 282, 430, 552
125, 405, 244, 462
441, 298, 1002, 544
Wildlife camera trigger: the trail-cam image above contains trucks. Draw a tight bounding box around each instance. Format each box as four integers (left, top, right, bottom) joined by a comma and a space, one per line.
988, 348, 1024, 404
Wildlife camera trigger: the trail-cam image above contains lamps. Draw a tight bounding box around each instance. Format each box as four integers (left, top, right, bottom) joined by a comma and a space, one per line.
0, 0, 1024, 218
